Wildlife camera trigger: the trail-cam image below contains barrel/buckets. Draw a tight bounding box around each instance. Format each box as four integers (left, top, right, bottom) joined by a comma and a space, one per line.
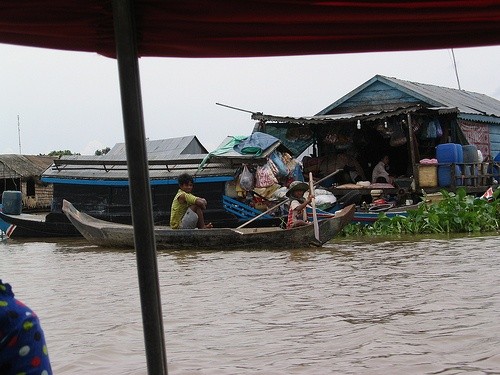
2, 191, 22, 214
418, 143, 489, 188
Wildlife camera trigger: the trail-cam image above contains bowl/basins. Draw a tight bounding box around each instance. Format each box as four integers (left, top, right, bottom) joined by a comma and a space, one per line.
369, 203, 394, 212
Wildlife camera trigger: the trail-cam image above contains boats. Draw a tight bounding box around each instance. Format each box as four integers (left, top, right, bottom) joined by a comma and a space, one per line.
62, 199, 355, 248
0, 155, 425, 237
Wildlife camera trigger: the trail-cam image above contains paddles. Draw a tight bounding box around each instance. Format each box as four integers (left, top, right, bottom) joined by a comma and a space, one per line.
309, 171, 323, 248
236, 168, 344, 229
363, 201, 370, 213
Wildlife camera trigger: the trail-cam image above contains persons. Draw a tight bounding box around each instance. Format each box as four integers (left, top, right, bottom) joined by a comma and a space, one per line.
286, 180, 315, 228
0, 279, 53, 375
372, 154, 394, 184
170, 174, 213, 229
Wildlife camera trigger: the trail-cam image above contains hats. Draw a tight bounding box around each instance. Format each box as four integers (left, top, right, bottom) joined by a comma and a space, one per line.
286, 181, 311, 197
